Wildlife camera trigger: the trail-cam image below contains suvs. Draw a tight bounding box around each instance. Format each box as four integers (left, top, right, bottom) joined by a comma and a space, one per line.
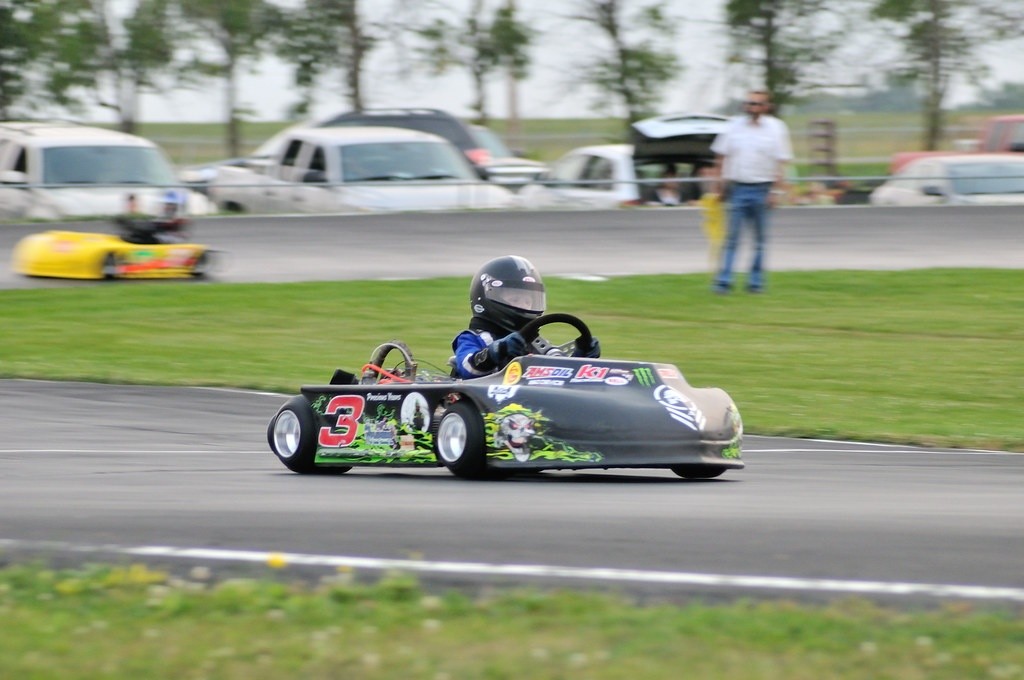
185, 105, 545, 185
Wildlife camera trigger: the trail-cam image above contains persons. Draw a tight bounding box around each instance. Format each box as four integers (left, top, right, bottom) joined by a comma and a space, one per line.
650, 161, 855, 208
450, 254, 601, 380
708, 81, 796, 296
121, 187, 190, 245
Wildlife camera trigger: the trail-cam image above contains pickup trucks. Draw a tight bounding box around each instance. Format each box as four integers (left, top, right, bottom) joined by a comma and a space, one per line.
891, 113, 1023, 170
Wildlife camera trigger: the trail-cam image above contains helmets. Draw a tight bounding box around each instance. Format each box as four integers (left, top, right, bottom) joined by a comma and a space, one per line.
469, 255, 546, 332
160, 188, 189, 218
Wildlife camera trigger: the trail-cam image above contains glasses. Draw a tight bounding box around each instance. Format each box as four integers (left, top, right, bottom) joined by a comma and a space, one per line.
744, 100, 771, 107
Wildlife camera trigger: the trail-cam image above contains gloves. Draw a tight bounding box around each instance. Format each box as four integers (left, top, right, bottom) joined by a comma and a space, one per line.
489, 332, 527, 366
572, 335, 601, 360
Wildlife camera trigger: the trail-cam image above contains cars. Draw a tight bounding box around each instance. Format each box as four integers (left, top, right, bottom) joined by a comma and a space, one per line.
870, 153, 1023, 208
0, 119, 187, 221
211, 123, 515, 213
516, 111, 743, 208
